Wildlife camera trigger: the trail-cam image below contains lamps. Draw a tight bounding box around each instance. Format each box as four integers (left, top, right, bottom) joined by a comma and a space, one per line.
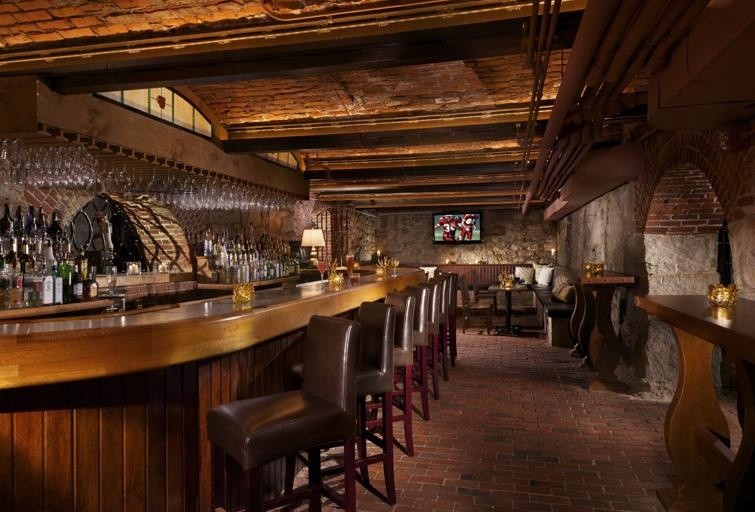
300, 228, 326, 268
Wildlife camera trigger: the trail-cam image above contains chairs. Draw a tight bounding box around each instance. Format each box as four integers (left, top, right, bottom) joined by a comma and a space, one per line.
460, 270, 497, 335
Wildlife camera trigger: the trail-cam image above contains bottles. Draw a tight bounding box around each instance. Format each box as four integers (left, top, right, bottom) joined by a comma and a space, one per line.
200, 218, 301, 284
0, 202, 100, 310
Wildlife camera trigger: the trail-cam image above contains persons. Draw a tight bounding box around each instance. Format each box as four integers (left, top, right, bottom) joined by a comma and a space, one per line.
457, 214, 479, 242
435, 214, 467, 242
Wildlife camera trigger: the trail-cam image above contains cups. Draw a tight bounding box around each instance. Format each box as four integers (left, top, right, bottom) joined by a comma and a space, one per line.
158, 260, 170, 273
232, 285, 256, 302
707, 284, 736, 306
707, 306, 736, 320
348, 273, 361, 288
583, 263, 604, 273
330, 273, 344, 285
125, 261, 142, 275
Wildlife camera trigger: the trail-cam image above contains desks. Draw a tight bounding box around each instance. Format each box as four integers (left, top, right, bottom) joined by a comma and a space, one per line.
569, 269, 636, 392
633, 294, 755, 511
488, 283, 530, 337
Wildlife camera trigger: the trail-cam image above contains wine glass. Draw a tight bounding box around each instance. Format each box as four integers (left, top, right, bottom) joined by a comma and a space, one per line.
390, 256, 400, 278
105, 265, 119, 311
316, 261, 329, 286
0, 139, 297, 211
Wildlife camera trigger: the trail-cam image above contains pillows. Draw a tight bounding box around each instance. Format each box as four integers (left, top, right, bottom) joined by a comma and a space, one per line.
514, 262, 554, 286
553, 274, 575, 302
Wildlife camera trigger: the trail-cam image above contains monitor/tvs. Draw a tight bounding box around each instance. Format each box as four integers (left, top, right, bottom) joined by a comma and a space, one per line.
432, 211, 483, 244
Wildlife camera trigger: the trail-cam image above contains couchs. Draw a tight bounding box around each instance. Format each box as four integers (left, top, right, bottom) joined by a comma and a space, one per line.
457, 263, 584, 346
